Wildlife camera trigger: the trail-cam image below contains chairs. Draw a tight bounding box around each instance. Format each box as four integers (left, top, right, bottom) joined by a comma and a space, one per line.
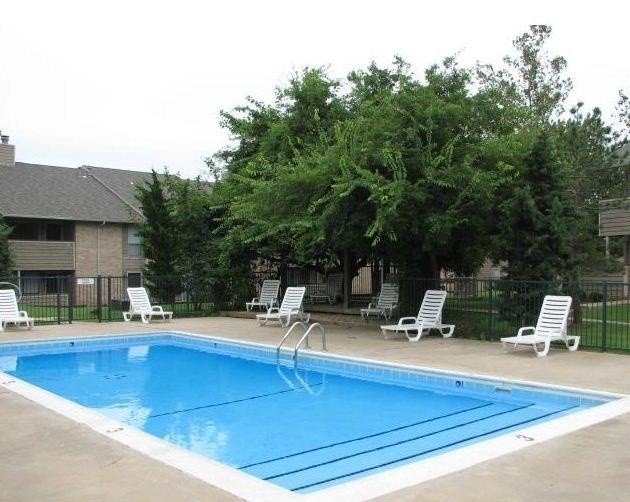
256, 287, 311, 327
246, 280, 280, 313
500, 295, 581, 356
123, 287, 173, 324
360, 283, 399, 321
380, 290, 461, 342
0, 289, 34, 331
